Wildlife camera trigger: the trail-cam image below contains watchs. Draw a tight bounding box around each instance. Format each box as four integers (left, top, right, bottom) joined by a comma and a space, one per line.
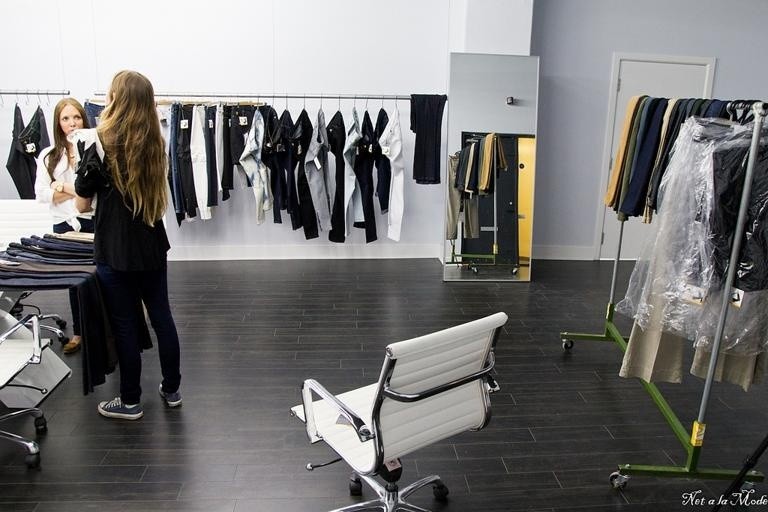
54, 180, 65, 193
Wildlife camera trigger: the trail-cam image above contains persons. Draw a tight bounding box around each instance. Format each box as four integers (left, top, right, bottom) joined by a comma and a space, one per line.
73, 68, 183, 420
34, 97, 97, 355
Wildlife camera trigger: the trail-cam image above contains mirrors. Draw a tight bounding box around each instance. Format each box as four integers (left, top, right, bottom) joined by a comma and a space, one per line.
442, 52, 540, 281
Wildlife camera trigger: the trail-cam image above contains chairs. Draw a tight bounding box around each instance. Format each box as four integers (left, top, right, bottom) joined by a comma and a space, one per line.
289, 310, 510, 512
0, 314, 54, 470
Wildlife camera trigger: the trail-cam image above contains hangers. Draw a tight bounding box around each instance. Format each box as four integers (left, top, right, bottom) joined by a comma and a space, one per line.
693, 99, 759, 148
86, 93, 267, 109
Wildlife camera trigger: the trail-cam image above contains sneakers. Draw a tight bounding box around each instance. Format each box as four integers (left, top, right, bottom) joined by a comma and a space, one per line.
98, 397, 144, 421
159, 382, 182, 408
64, 335, 80, 353
